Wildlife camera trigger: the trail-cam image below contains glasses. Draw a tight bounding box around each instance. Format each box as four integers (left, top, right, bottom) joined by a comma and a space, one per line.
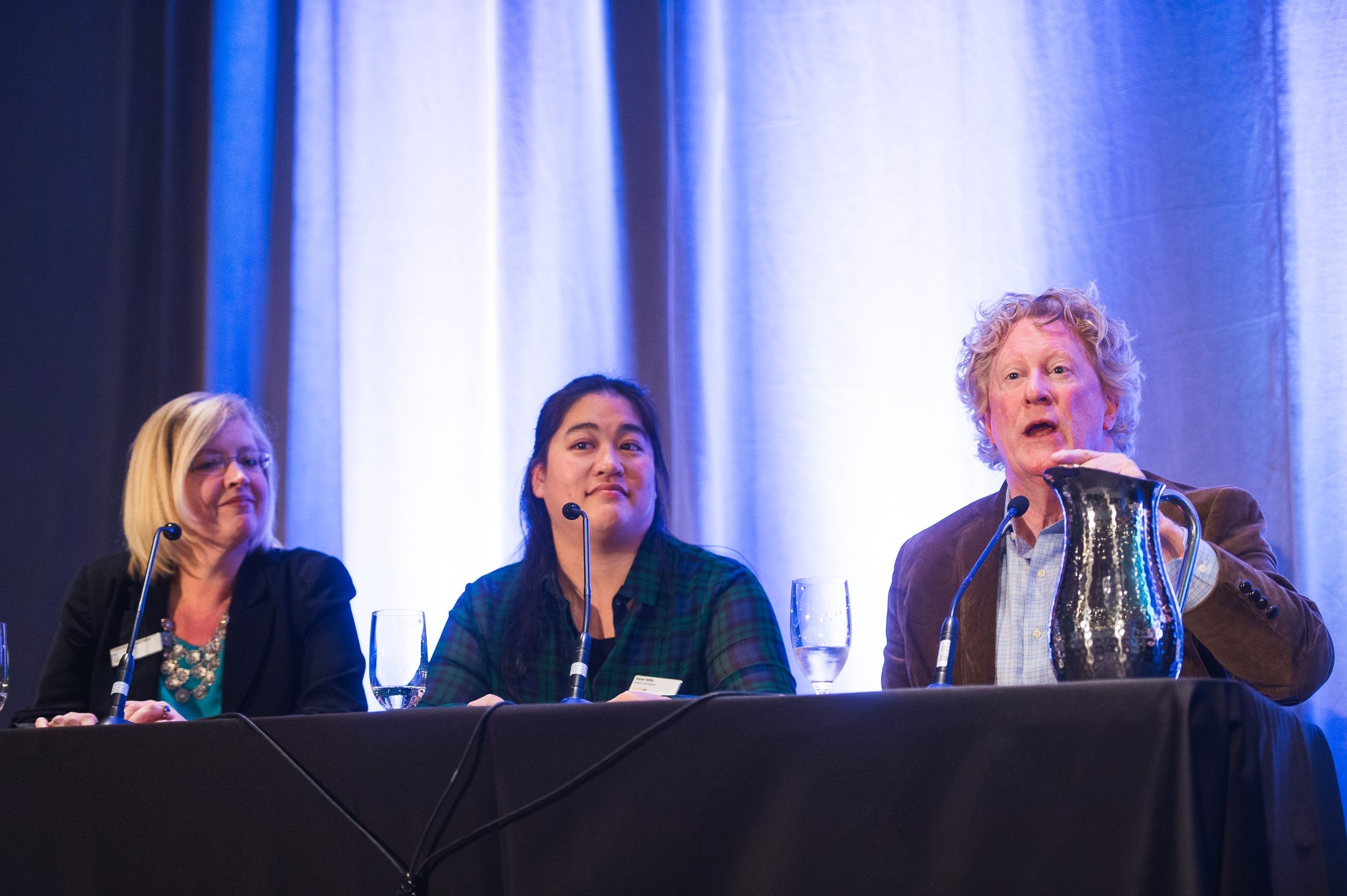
190, 448, 272, 476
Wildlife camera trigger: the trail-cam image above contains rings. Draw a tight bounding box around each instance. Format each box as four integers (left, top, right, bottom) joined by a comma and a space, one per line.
159, 706, 174, 710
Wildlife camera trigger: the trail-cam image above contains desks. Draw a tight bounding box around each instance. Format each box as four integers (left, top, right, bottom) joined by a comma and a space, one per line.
0, 674, 1347, 896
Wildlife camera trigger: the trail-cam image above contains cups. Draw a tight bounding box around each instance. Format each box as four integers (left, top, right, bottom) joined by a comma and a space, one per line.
369, 610, 428, 710
0, 622, 10, 711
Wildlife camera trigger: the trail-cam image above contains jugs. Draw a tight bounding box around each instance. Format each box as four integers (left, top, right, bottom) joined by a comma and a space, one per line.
1042, 466, 1200, 682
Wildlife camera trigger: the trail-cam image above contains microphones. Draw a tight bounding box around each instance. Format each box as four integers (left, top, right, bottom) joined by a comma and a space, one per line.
561, 502, 592, 705
96, 524, 182, 725
928, 494, 1031, 689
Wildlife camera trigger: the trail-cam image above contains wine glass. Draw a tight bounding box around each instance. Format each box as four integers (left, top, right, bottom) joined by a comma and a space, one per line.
790, 577, 851, 694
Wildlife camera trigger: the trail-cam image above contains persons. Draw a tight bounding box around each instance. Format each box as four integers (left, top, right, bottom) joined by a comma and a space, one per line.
410, 376, 797, 706
880, 277, 1335, 709
29, 391, 369, 728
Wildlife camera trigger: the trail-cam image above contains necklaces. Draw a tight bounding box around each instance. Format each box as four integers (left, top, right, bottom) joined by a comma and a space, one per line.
153, 601, 230, 704
